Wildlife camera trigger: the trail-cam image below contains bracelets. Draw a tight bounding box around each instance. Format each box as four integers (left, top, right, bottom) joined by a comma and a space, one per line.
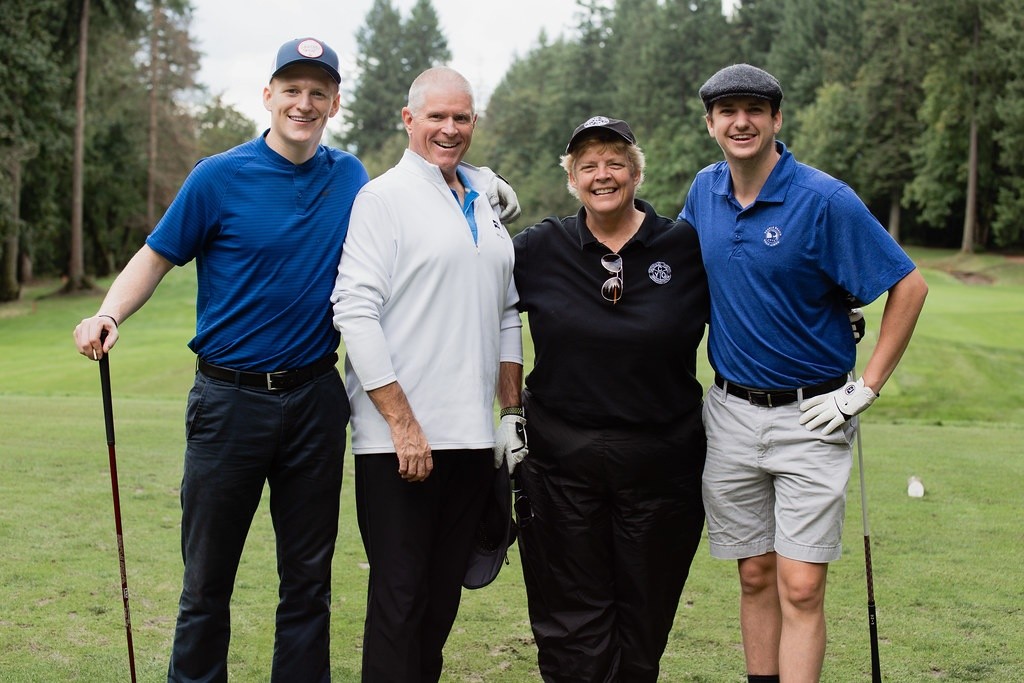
500, 406, 523, 419
99, 314, 118, 327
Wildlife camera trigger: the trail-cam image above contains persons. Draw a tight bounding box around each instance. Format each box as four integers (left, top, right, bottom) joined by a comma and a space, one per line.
676, 65, 929, 683
330, 67, 522, 683
74, 38, 522, 683
508, 115, 865, 683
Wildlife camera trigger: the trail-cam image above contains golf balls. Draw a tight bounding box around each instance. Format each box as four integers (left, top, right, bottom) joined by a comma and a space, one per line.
907, 482, 924, 497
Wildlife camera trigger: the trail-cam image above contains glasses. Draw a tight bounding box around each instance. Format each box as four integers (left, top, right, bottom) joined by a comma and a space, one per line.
601, 251, 623, 304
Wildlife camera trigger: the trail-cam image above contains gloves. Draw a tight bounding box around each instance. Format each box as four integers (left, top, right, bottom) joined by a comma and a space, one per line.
481, 166, 521, 224
848, 308, 865, 344
798, 377, 880, 436
494, 414, 529, 475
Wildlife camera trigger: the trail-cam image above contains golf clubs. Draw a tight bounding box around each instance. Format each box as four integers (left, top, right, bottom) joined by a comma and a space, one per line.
850, 368, 883, 683
98, 329, 137, 682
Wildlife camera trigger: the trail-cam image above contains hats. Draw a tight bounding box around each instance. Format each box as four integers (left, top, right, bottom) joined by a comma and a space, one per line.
269, 37, 341, 94
463, 453, 519, 590
699, 63, 783, 113
566, 116, 637, 155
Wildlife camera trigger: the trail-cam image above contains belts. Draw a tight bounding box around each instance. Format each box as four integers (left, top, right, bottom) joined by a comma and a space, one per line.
714, 371, 847, 407
198, 352, 339, 390
